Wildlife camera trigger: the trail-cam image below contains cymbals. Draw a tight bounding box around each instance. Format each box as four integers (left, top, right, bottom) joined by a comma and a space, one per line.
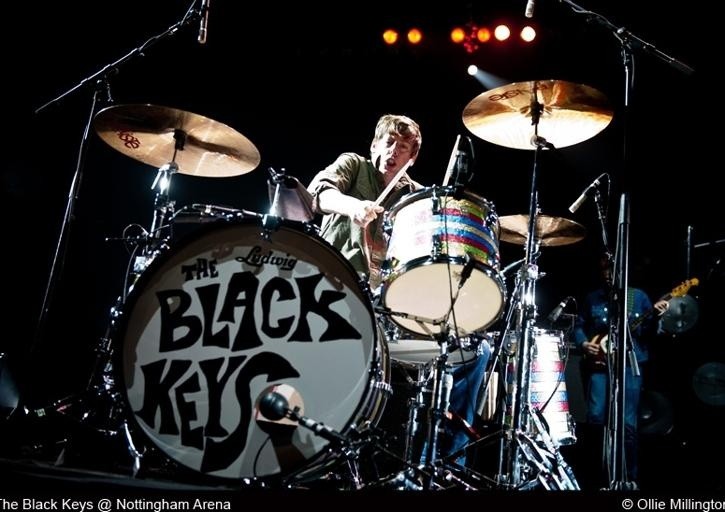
93, 103, 261, 178
462, 81, 614, 150
498, 213, 586, 247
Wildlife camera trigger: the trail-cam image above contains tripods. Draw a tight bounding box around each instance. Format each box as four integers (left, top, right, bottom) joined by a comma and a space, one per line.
370, 155, 582, 492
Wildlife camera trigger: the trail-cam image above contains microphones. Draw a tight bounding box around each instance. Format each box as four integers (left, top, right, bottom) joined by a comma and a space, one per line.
256, 388, 292, 427
564, 170, 610, 214
524, 0, 537, 19
195, 2, 210, 44
685, 223, 694, 281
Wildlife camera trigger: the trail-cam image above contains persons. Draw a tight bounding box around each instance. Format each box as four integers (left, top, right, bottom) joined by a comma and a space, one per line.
569, 256, 670, 492
306, 112, 490, 470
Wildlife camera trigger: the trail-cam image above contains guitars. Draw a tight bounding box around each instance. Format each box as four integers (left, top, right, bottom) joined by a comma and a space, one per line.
583, 277, 699, 366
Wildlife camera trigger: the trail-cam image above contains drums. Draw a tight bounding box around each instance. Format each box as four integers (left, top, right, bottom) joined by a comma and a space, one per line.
495, 330, 577, 448
381, 188, 508, 340
385, 324, 493, 369
115, 216, 392, 481
